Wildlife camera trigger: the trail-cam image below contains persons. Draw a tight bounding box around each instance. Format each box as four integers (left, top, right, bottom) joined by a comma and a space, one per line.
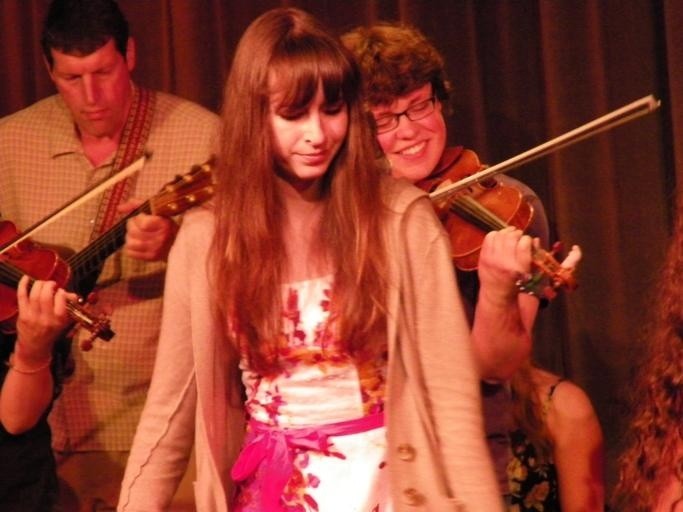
528, 359, 606, 511
0, 275, 75, 511
606, 228, 683, 511
340, 19, 553, 509
0, 0, 235, 512
112, 4, 509, 512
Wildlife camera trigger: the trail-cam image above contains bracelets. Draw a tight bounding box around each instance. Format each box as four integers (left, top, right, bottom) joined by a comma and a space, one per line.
8, 349, 53, 373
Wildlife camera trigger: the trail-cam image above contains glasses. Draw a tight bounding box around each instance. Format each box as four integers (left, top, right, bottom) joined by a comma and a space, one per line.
369, 86, 437, 135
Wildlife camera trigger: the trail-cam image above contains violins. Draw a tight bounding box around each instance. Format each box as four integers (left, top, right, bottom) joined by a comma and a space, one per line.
0, 220, 115, 349
414, 149, 581, 308
